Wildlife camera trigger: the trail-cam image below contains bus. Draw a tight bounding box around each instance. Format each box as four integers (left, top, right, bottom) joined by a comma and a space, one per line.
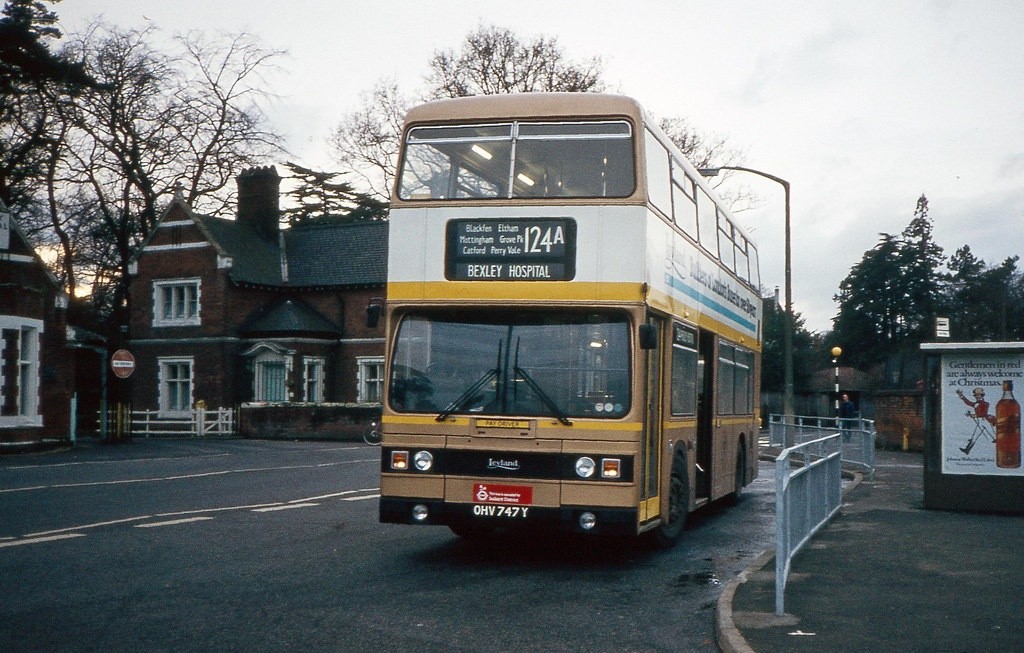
363, 93, 765, 543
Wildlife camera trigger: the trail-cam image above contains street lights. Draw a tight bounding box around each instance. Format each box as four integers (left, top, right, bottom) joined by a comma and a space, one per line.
830, 346, 842, 444
695, 165, 794, 456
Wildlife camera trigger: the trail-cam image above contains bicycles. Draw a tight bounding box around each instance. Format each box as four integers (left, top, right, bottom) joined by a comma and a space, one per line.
363, 416, 382, 446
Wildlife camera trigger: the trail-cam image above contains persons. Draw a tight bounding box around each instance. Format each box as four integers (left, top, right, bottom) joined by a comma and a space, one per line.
839, 393, 855, 443
417, 336, 471, 408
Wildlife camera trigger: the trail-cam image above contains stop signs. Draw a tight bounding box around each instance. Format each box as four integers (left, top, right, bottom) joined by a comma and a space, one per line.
111, 349, 136, 379
